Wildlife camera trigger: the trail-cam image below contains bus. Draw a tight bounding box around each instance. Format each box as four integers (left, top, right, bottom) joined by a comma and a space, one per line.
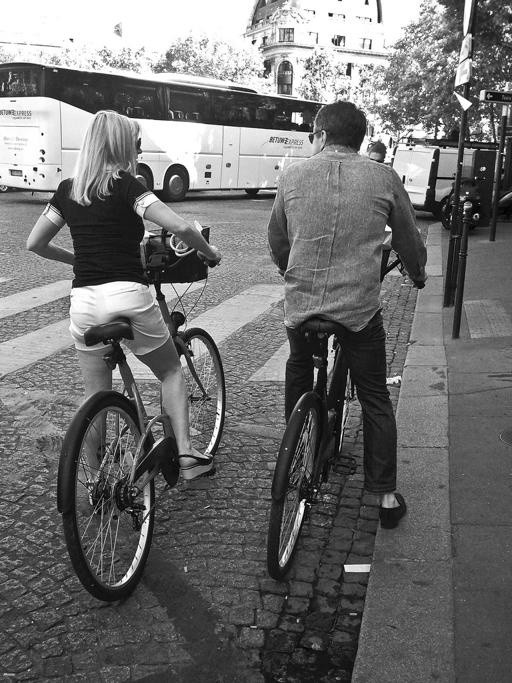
0, 62, 328, 201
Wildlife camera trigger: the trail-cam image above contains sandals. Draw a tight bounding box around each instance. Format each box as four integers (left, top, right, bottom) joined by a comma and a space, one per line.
178, 448, 214, 479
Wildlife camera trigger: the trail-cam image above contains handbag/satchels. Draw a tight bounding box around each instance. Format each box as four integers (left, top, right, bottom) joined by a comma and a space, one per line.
139, 220, 204, 282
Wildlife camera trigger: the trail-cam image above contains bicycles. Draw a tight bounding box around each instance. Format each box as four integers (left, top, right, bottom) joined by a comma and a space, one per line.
267, 245, 401, 579
56, 224, 226, 602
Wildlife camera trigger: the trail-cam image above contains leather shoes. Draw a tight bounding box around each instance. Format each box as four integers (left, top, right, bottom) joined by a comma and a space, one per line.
379, 492, 406, 530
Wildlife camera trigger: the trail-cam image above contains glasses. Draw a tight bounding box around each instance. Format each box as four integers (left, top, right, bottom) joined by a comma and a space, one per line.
136, 137, 141, 148
309, 129, 321, 144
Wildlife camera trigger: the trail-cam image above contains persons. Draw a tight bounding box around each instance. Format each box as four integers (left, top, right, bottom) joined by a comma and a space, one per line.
26, 110, 213, 513
264, 103, 426, 530
367, 141, 387, 163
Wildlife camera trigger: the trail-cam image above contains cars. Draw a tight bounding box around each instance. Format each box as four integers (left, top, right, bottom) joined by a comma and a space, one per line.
391, 146, 494, 218
